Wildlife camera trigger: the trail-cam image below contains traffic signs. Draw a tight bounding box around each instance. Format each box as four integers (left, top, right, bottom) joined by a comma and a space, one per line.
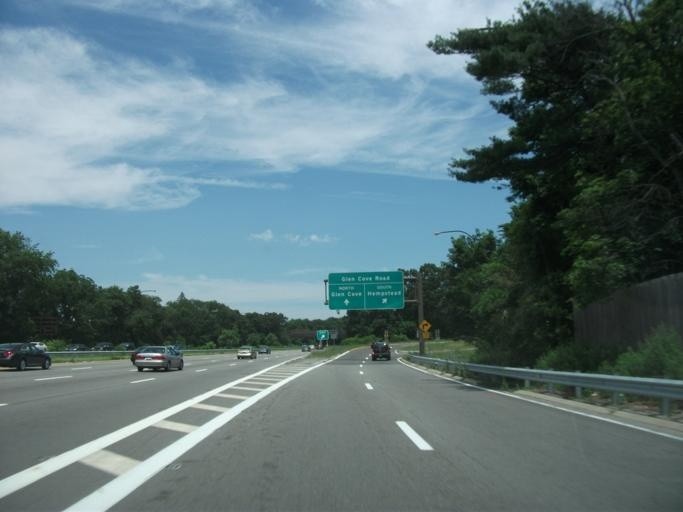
328, 271, 405, 310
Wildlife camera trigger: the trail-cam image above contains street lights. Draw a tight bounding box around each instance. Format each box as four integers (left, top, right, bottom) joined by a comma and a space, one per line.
433, 230, 475, 242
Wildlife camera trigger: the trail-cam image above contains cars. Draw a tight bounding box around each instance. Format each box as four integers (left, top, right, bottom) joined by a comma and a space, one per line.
0, 342, 51, 371
237, 345, 271, 359
302, 344, 315, 352
64, 342, 184, 372
371, 341, 392, 361
